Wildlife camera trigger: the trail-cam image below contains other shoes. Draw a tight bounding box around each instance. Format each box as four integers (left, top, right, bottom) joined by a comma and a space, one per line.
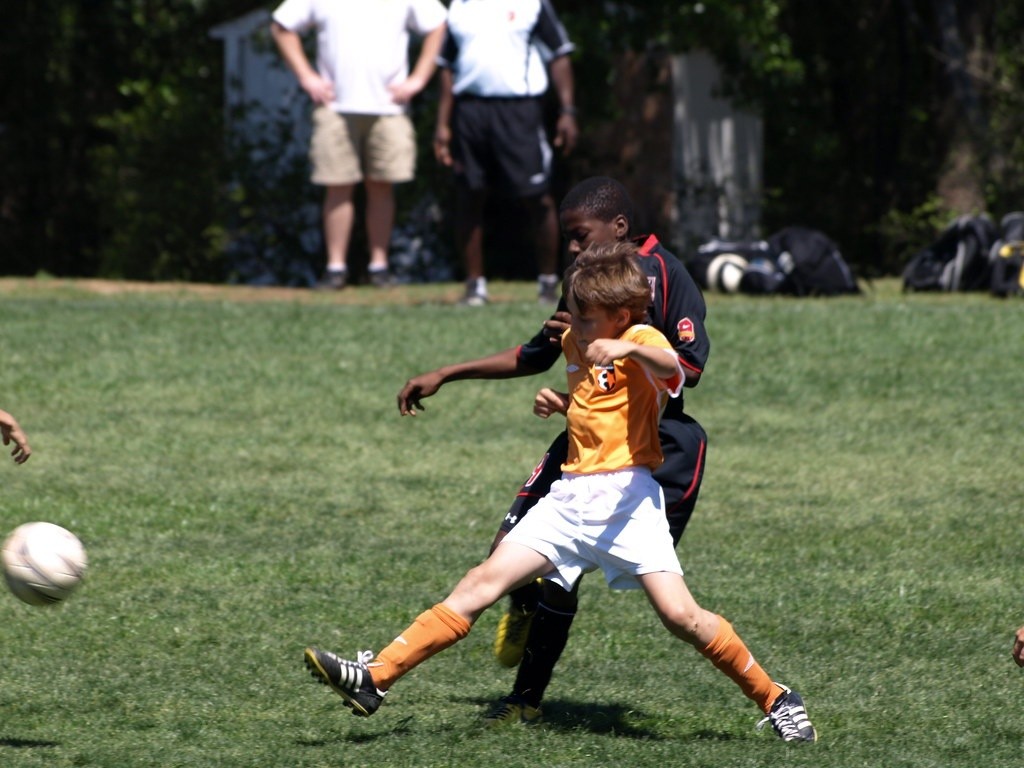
454, 276, 490, 312
365, 268, 390, 290
318, 267, 346, 292
534, 273, 561, 309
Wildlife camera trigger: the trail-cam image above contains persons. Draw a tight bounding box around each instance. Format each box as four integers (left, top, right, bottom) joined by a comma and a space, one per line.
396, 176, 710, 724
269, 0, 448, 288
0, 410, 31, 465
433, 0, 580, 306
305, 245, 818, 748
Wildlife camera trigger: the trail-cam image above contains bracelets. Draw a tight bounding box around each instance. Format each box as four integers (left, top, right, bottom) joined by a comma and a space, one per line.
561, 105, 577, 115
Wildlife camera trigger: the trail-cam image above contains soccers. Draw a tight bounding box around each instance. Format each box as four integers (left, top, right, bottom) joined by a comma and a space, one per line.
0, 519, 92, 606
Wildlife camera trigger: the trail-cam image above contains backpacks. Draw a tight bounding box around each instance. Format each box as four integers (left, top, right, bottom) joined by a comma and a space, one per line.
902, 211, 996, 297
686, 228, 876, 298
981, 211, 1024, 300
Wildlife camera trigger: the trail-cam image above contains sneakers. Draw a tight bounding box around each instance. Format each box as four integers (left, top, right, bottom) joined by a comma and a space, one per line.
756, 682, 818, 747
470, 692, 545, 737
305, 645, 389, 717
495, 576, 543, 668
